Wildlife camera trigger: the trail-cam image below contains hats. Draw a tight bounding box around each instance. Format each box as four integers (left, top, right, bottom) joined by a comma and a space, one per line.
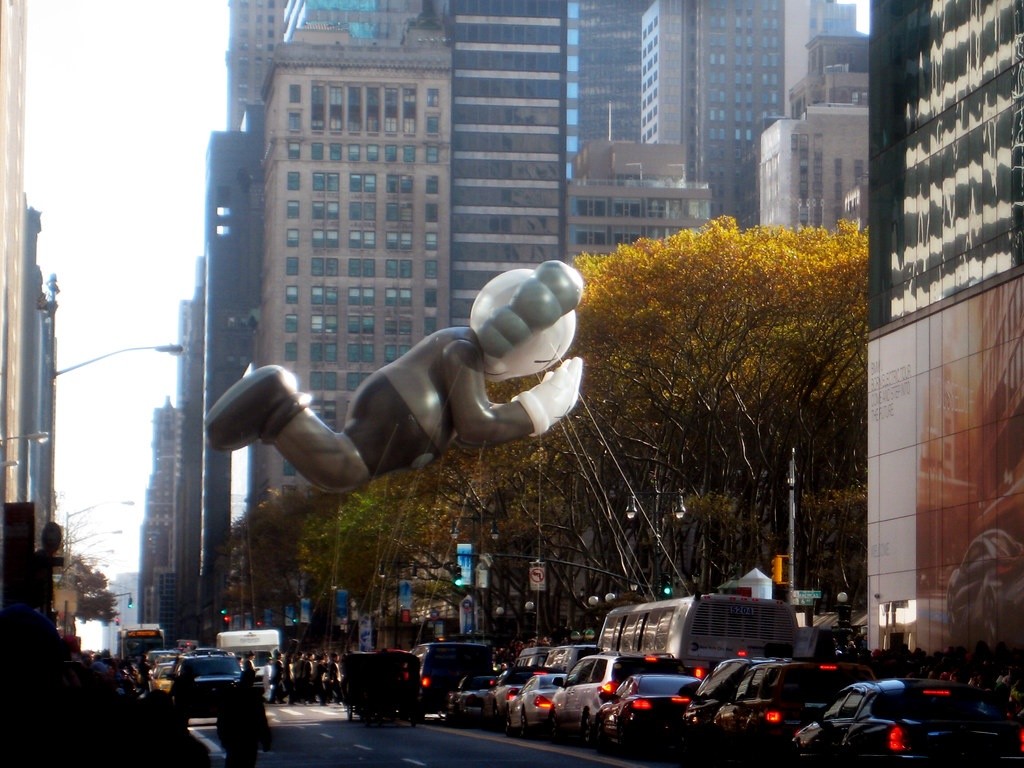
0, 603, 65, 686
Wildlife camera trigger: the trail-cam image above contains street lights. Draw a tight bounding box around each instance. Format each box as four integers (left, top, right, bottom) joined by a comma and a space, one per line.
62, 499, 135, 638
34, 271, 185, 551
623, 475, 688, 601
449, 504, 502, 644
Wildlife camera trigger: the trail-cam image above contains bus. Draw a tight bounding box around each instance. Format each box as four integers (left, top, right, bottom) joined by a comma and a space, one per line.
115, 623, 166, 659
596, 588, 798, 681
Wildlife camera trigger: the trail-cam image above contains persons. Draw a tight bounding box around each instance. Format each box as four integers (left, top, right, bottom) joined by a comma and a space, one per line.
833, 631, 1024, 724
495, 636, 569, 676
263, 649, 348, 706
0, 604, 273, 768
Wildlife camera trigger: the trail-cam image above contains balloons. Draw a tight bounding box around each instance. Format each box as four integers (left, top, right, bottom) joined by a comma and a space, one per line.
206, 259, 584, 494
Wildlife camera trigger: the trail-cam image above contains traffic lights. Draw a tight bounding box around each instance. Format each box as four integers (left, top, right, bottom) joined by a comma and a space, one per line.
660, 572, 674, 601
128, 598, 133, 608
770, 557, 783, 583
115, 617, 119, 626
451, 564, 462, 588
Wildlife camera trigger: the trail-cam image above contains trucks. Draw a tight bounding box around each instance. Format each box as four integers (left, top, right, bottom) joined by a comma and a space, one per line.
216, 628, 282, 690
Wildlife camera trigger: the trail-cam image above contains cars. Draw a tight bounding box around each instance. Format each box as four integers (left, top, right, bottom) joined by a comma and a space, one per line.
336, 639, 1024, 768
144, 638, 242, 726
